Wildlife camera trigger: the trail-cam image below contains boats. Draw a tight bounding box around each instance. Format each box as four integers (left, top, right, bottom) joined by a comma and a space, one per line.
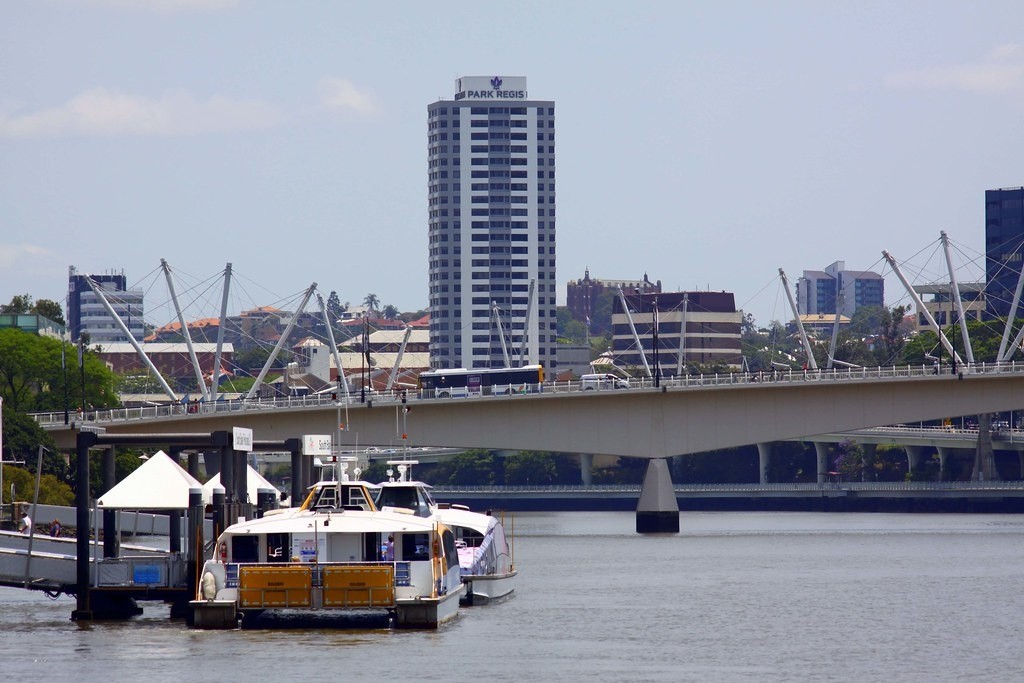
185, 405, 463, 628
370, 406, 517, 608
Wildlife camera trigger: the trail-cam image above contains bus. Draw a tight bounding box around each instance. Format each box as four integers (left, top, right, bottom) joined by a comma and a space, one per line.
417, 364, 543, 398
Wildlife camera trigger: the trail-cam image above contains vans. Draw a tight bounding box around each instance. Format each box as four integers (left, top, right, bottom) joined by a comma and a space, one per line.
992, 421, 1009, 431
578, 374, 631, 391
